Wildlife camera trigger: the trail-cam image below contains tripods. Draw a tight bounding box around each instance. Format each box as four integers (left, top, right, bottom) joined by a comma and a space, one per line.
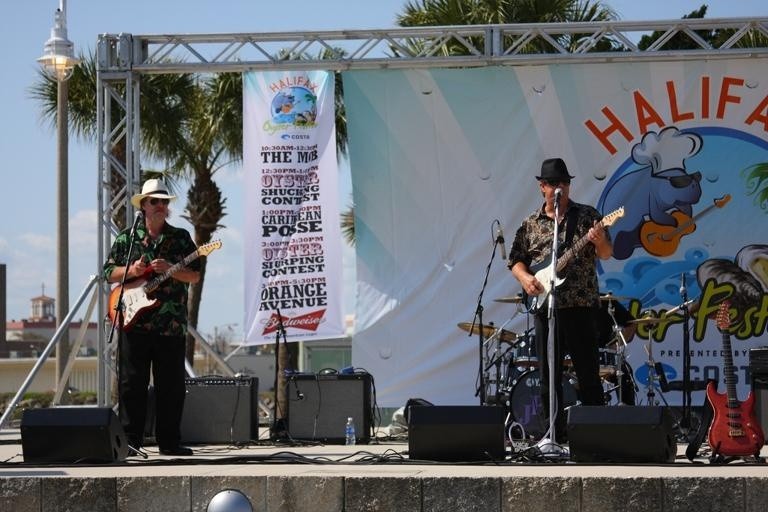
235, 332, 322, 446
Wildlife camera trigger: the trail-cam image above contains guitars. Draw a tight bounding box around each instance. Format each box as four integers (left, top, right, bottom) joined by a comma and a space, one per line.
109, 240, 222, 329
707, 300, 764, 456
640, 194, 731, 256
524, 206, 625, 314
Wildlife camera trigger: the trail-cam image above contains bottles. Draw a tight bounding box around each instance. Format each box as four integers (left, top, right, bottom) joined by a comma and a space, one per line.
345, 417, 355, 446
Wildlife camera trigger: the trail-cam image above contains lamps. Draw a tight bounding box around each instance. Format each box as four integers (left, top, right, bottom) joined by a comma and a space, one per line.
562, 402, 679, 468
16, 403, 134, 468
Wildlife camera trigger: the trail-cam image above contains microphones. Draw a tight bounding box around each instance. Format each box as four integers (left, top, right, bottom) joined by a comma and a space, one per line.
554, 188, 562, 208
680, 273, 686, 294
129, 210, 144, 237
496, 225, 506, 260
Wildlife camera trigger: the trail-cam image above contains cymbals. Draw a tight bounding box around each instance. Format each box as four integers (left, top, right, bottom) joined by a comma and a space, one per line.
458, 322, 518, 340
599, 296, 625, 301
627, 317, 672, 323
492, 296, 524, 303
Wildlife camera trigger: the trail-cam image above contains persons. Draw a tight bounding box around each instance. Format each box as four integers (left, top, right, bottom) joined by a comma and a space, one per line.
506, 157, 612, 454
101, 179, 203, 457
568, 294, 638, 408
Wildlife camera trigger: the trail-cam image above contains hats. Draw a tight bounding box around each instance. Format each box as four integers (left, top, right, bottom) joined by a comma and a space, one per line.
131, 179, 176, 208
536, 159, 574, 180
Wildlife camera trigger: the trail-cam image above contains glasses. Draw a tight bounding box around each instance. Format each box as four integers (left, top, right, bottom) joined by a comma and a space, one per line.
150, 198, 169, 204
549, 180, 570, 186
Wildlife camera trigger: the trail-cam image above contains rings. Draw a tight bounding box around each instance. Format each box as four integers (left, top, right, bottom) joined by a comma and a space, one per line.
592, 237, 598, 241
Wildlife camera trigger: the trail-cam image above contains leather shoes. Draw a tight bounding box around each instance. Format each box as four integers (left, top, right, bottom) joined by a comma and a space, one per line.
160, 447, 193, 456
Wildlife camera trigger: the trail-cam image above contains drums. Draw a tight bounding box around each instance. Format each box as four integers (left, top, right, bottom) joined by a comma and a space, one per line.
505, 335, 616, 442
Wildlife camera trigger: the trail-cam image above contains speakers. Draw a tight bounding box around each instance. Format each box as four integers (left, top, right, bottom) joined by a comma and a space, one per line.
567, 406, 678, 464
21, 408, 129, 465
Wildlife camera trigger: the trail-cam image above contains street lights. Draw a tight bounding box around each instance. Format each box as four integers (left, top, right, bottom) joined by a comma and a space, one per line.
35, 0, 82, 406
